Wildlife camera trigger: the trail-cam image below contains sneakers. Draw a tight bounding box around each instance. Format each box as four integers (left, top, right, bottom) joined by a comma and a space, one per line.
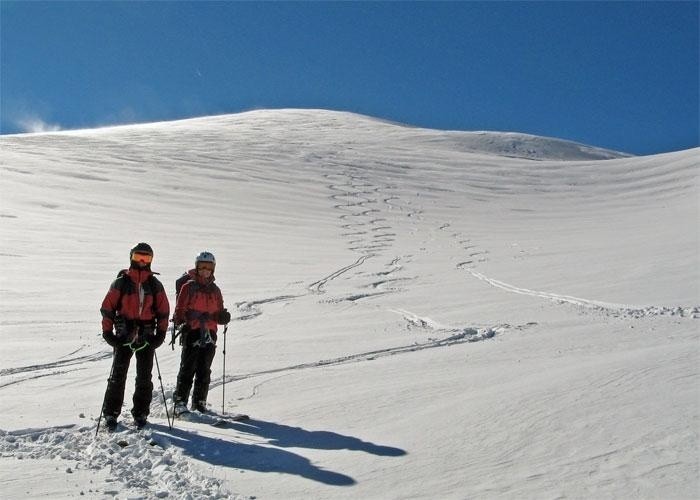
173, 402, 190, 418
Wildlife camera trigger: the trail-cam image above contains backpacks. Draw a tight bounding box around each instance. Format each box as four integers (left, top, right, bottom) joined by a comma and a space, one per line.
175, 273, 192, 294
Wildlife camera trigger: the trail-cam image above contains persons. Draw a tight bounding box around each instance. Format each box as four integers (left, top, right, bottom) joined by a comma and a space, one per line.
171, 249, 231, 420
100, 241, 170, 432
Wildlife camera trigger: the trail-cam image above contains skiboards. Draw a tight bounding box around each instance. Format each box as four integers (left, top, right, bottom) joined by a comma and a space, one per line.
115, 432, 157, 448
178, 414, 249, 426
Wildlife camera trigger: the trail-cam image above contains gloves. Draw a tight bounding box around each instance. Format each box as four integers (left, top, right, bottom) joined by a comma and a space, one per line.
151, 334, 165, 349
102, 331, 118, 346
188, 309, 200, 319
218, 309, 230, 324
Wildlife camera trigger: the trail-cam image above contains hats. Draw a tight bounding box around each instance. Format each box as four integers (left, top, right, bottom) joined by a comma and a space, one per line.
130, 242, 153, 257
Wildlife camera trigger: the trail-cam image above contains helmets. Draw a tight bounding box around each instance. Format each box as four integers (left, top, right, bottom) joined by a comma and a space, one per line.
195, 251, 216, 265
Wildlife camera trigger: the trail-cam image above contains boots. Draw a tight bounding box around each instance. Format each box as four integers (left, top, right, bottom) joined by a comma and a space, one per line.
133, 413, 147, 426
192, 400, 212, 414
104, 409, 119, 428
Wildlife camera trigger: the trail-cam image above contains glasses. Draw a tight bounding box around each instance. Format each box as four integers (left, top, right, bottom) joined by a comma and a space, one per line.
131, 253, 152, 263
197, 262, 213, 270
